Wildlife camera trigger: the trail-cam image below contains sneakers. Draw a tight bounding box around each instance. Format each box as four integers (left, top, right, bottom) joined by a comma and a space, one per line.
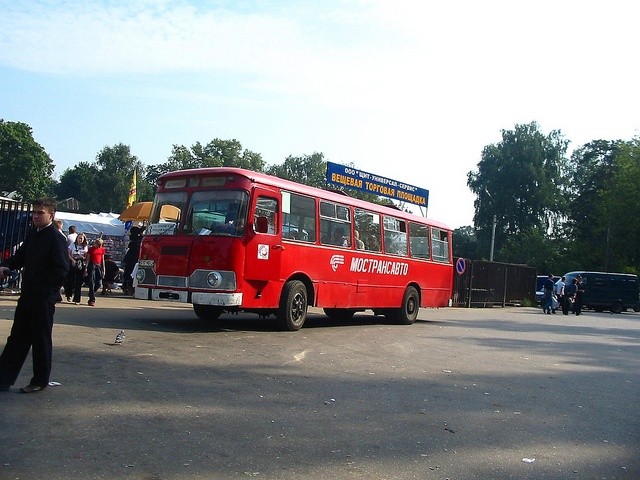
547, 312, 551, 314
75, 301, 79, 304
67, 296, 71, 301
553, 311, 556, 314
88, 301, 94, 306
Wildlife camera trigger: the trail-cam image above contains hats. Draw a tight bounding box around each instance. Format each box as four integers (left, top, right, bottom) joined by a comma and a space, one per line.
573, 279, 578, 282
577, 275, 582, 278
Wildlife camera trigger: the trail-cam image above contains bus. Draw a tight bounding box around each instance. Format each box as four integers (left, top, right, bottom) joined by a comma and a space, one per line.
134, 168, 454, 332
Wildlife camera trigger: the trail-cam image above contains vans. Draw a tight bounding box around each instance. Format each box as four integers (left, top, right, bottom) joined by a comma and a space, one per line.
554, 268, 636, 314
536, 275, 560, 302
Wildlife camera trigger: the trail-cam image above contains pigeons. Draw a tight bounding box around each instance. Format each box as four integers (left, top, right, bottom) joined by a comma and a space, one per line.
114, 330, 126, 346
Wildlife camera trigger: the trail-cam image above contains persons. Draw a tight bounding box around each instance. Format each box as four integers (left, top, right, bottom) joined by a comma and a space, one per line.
0, 197, 70, 393
576, 277, 585, 316
543, 276, 557, 314
56, 220, 66, 239
349, 229, 365, 252
334, 228, 347, 246
67, 232, 88, 305
569, 278, 578, 314
216, 203, 256, 231
101, 254, 124, 295
544, 273, 558, 315
83, 238, 105, 306
66, 226, 78, 255
368, 234, 380, 251
557, 276, 569, 315
572, 274, 583, 314
287, 230, 298, 241
124, 226, 142, 289
385, 238, 397, 252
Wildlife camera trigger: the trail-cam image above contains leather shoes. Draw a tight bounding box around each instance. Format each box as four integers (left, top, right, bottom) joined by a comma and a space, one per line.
544, 308, 546, 314
20, 385, 42, 392
0, 386, 10, 390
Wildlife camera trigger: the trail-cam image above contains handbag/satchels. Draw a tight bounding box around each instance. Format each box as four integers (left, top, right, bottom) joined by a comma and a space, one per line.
74, 241, 86, 274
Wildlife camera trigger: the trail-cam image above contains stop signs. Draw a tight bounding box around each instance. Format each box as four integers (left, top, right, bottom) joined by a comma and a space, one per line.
456, 258, 466, 275
